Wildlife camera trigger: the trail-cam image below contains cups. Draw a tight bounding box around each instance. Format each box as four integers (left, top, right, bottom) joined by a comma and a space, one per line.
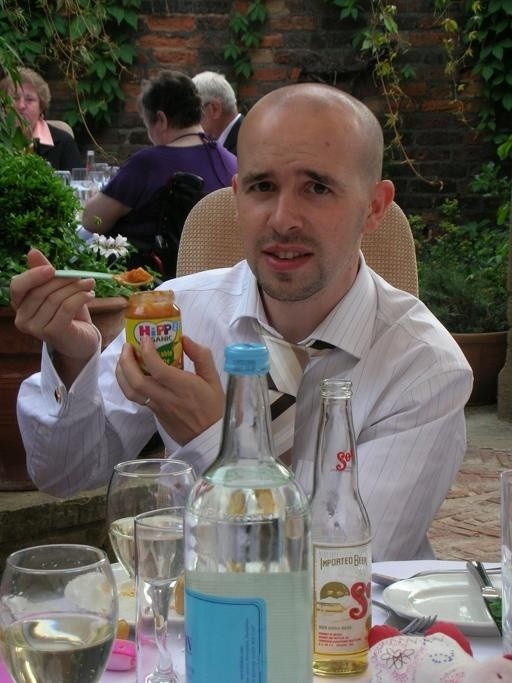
53, 150, 120, 212
0, 543, 119, 683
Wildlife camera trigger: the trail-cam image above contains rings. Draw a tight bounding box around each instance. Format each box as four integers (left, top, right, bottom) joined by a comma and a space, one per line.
139, 397, 151, 407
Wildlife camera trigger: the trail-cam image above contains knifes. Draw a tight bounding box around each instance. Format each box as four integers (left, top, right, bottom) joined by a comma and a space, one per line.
466, 559, 503, 638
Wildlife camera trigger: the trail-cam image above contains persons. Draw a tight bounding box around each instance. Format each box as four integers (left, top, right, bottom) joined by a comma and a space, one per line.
80, 71, 239, 290
0, 65, 86, 181
191, 71, 247, 155
9, 84, 476, 564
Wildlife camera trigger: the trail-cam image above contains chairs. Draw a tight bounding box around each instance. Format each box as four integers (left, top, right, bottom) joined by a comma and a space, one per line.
174, 186, 419, 303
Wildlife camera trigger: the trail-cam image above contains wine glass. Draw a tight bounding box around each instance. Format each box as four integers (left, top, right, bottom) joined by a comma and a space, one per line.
104, 457, 199, 683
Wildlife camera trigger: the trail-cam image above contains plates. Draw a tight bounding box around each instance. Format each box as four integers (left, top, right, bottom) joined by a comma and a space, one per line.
62, 561, 185, 633
382, 573, 503, 637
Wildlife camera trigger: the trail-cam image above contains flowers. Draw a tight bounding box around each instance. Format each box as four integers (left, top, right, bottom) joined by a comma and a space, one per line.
0, 147, 130, 301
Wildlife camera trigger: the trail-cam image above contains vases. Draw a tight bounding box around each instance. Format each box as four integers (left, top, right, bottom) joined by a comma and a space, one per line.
0, 293, 130, 493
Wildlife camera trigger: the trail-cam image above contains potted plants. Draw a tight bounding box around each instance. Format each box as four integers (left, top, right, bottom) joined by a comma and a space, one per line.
414, 198, 509, 405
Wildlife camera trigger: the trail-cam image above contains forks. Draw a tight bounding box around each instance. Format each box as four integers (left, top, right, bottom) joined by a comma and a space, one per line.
372, 599, 439, 636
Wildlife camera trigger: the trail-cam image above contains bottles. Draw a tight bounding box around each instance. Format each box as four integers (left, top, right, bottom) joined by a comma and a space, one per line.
308, 377, 375, 680
183, 341, 314, 683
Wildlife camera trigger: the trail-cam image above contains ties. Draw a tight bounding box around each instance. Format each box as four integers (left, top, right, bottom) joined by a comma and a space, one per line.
254, 323, 334, 471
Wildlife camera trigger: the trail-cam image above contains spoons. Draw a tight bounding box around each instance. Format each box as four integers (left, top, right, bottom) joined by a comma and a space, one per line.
54, 269, 154, 286
